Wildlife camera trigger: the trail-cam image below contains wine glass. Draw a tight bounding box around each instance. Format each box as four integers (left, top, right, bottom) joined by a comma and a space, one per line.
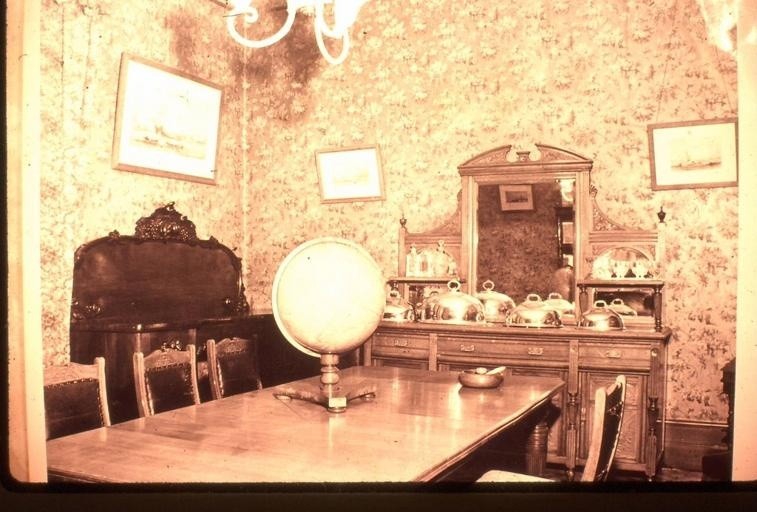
611, 260, 649, 281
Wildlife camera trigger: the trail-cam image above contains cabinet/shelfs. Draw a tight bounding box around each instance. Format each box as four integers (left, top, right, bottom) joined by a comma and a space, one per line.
577, 340, 655, 469
369, 332, 429, 371
438, 335, 570, 462
576, 230, 666, 332
386, 221, 458, 317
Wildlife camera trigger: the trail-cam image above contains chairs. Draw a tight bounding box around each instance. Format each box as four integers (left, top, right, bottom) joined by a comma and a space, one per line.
132, 344, 200, 417
43, 357, 111, 440
206, 334, 263, 400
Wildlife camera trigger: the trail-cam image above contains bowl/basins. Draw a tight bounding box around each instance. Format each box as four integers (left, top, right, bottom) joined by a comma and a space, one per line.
459, 368, 504, 388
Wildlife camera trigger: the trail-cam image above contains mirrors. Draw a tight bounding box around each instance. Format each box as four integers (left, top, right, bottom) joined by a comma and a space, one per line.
458, 142, 594, 305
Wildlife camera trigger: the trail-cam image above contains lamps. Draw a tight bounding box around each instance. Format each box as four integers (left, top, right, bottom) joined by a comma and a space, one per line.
226, 0, 367, 64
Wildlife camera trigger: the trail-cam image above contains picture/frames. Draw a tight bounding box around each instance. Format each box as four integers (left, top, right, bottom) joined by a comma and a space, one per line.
499, 184, 537, 212
646, 117, 739, 191
314, 144, 387, 204
111, 53, 224, 186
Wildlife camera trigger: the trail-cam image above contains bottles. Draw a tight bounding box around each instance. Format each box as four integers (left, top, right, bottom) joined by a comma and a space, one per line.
403, 240, 449, 279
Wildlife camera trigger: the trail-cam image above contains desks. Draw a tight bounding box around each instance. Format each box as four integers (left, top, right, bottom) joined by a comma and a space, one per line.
91, 313, 296, 424
47, 365, 565, 483
